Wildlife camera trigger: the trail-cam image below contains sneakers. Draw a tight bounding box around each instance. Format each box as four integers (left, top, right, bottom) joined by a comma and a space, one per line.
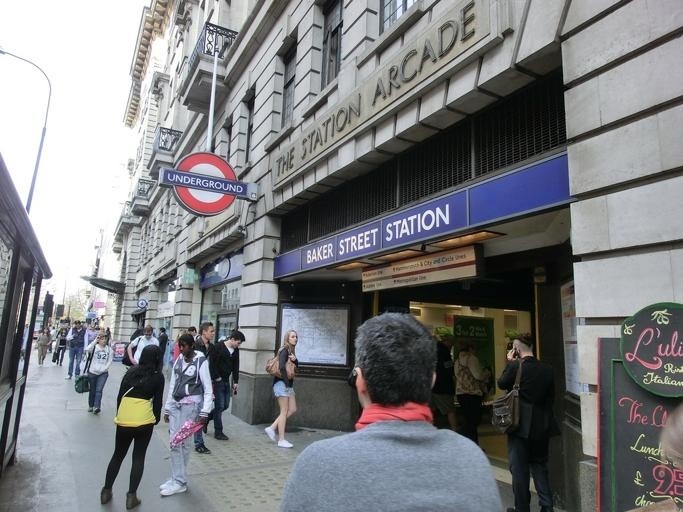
203, 417, 228, 440
196, 446, 210, 454
265, 427, 293, 447
88, 407, 100, 413
160, 481, 187, 496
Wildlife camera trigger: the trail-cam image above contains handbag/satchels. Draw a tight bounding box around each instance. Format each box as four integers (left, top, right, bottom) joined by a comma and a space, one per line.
492, 390, 519, 434
122, 338, 140, 366
266, 345, 296, 380
76, 376, 89, 393
459, 354, 485, 394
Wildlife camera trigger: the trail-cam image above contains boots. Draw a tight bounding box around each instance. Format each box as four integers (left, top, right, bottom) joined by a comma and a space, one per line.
101, 488, 112, 504
127, 492, 141, 509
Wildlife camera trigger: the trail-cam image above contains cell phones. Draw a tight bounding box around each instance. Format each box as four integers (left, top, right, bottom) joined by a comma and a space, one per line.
514, 346, 520, 355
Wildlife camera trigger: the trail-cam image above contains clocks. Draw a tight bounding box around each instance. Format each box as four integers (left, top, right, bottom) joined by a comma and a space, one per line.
135, 298, 148, 309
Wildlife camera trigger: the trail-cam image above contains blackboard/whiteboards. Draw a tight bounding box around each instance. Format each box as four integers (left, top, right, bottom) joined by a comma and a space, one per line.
596, 301, 683, 512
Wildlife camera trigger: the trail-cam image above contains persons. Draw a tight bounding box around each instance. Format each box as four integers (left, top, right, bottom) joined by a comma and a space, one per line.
264, 330, 298, 448
453, 344, 490, 445
278, 312, 504, 511
19, 324, 28, 359
428, 333, 455, 430
496, 333, 561, 512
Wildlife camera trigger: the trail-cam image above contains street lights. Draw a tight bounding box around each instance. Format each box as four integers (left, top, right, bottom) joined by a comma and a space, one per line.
0, 46, 54, 216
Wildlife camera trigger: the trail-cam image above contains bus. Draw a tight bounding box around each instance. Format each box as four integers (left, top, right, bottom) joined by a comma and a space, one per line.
57, 315, 74, 328
33, 304, 50, 338
